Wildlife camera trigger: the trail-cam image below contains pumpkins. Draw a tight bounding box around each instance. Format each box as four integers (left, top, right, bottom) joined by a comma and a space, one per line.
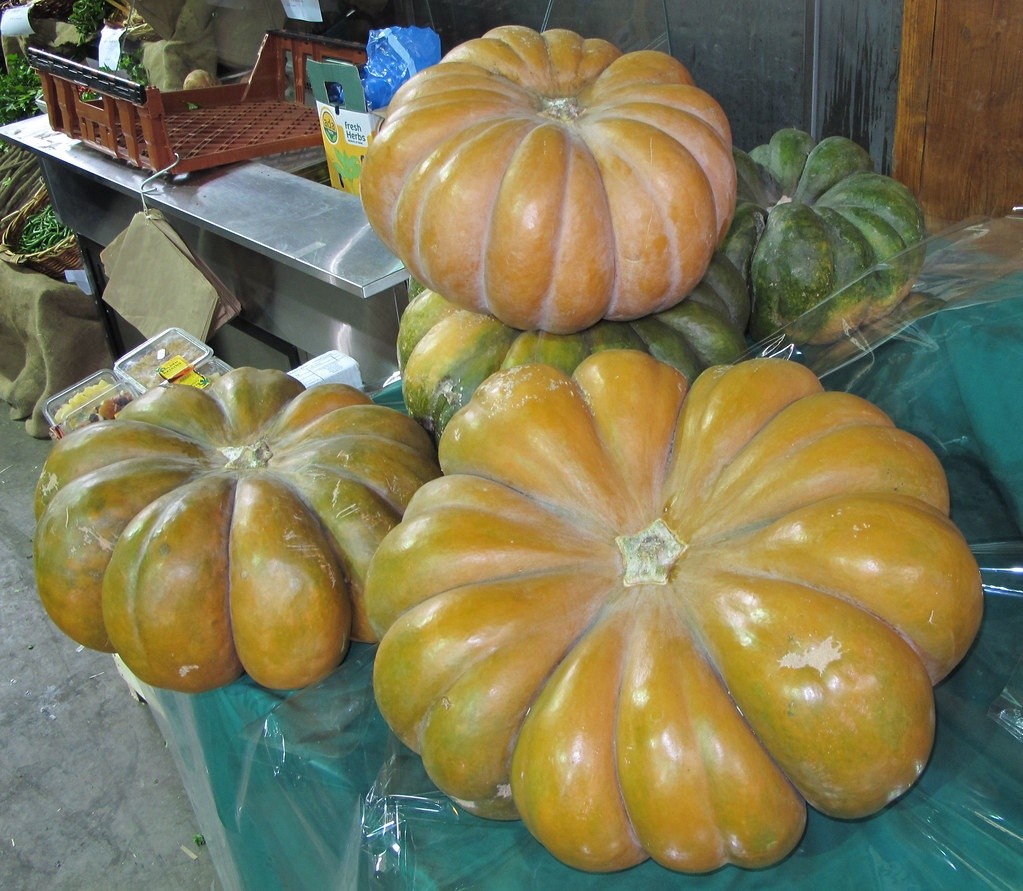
35, 25, 989, 878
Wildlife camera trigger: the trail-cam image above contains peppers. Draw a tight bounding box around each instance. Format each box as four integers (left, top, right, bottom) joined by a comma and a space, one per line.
14, 199, 74, 255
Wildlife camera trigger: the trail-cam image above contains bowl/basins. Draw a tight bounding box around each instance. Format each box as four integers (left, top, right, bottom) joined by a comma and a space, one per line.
41, 325, 235, 436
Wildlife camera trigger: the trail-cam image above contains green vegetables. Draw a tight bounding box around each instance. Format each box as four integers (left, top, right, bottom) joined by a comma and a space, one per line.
0, 50, 48, 121
